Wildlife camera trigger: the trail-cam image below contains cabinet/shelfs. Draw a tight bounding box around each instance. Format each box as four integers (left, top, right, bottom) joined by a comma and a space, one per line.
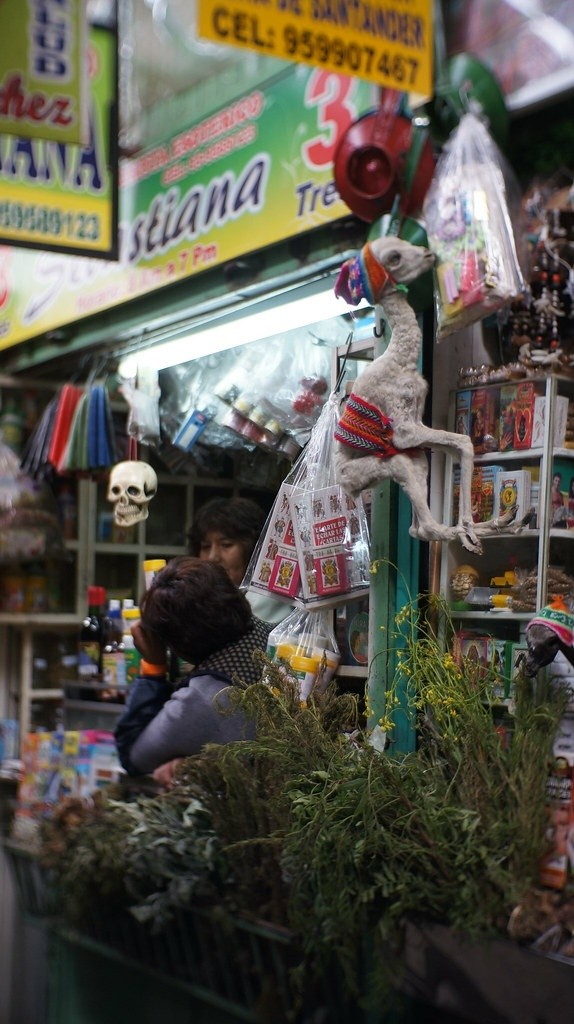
0, 374, 288, 738
435, 373, 574, 710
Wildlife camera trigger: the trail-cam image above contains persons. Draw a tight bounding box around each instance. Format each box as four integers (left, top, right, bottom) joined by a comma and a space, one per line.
112, 498, 298, 777
551, 472, 567, 527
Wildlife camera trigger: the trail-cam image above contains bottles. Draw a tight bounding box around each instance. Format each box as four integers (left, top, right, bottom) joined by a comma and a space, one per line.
77, 585, 141, 684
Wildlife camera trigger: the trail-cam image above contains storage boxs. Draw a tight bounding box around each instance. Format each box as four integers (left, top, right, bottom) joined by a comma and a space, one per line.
531, 395, 569, 448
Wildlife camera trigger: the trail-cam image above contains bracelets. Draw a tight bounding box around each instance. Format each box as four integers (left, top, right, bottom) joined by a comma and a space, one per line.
137, 659, 167, 677
